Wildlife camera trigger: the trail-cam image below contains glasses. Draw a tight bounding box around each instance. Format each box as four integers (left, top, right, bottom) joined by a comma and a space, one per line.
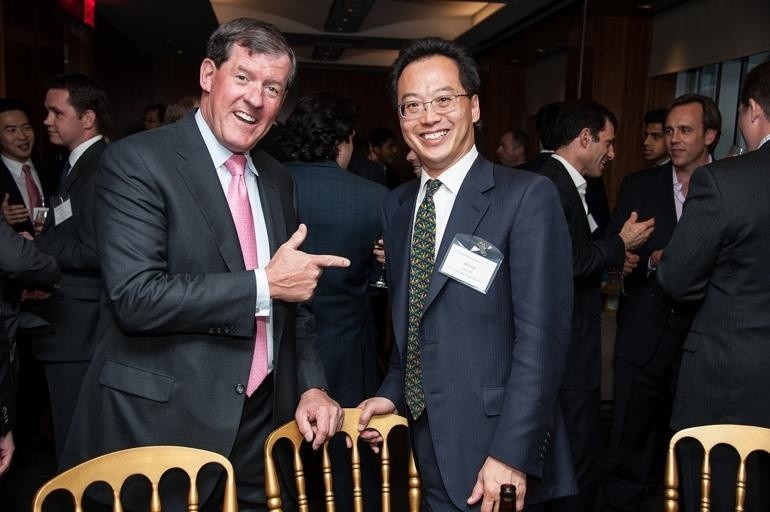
397, 95, 469, 119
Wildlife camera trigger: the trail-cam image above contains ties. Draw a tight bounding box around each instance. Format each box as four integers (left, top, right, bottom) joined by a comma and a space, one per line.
404, 179, 443, 422
58, 158, 71, 192
223, 154, 269, 399
22, 165, 46, 237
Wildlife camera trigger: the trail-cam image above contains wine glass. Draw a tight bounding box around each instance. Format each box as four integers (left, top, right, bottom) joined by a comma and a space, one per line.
370, 226, 385, 288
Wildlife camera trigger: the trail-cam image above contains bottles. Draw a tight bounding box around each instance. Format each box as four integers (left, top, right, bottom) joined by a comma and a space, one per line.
494, 481, 520, 511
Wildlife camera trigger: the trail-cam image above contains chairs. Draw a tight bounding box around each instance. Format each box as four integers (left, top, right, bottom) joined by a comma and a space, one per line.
665, 424, 770, 512
32, 445, 238, 512
264, 408, 422, 512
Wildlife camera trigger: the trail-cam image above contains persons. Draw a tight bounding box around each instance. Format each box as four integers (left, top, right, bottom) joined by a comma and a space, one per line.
346, 37, 581, 512
53, 19, 346, 512
1, 56, 769, 512
